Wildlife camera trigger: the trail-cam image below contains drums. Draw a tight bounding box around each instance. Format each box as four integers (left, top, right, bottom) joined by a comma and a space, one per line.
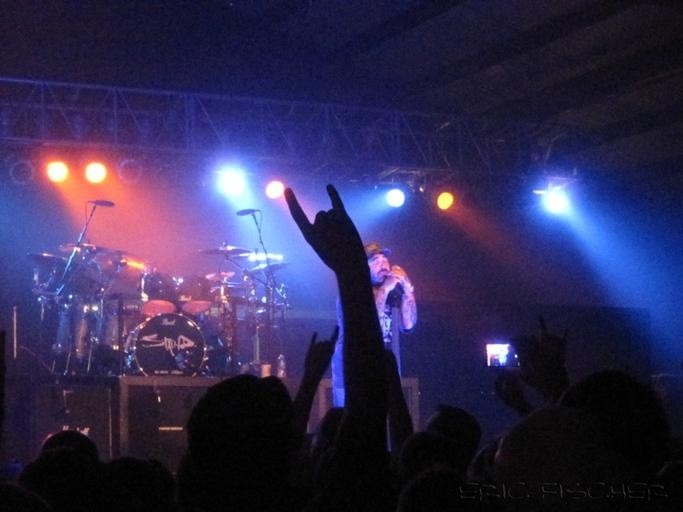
173, 273, 213, 316
51, 302, 101, 361
136, 271, 180, 316
124, 313, 206, 377
105, 299, 143, 351
99, 248, 148, 298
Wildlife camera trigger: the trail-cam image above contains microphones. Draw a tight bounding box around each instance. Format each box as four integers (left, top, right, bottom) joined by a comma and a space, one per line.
235, 209, 256, 217
386, 273, 403, 296
93, 200, 116, 208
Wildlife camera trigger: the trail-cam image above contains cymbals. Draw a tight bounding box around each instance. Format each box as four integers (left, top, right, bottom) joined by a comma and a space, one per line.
198, 245, 253, 255
26, 251, 66, 262
246, 262, 285, 274
205, 270, 235, 281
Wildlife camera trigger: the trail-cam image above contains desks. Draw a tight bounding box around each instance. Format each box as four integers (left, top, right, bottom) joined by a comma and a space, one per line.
116, 375, 308, 455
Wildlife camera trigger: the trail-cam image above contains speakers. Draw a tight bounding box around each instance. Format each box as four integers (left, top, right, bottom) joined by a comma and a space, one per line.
119, 374, 221, 465
27, 377, 118, 464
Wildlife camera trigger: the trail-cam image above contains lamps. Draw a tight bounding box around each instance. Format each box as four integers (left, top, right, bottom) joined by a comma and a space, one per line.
386, 185, 417, 208
418, 182, 453, 211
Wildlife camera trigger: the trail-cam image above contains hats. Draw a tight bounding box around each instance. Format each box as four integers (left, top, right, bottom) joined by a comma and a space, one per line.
364, 243, 392, 259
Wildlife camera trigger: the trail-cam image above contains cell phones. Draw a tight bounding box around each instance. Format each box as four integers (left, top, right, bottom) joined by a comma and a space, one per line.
479, 337, 517, 368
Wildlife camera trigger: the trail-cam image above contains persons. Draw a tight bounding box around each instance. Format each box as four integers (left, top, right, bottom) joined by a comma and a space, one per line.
0, 184, 683, 512
